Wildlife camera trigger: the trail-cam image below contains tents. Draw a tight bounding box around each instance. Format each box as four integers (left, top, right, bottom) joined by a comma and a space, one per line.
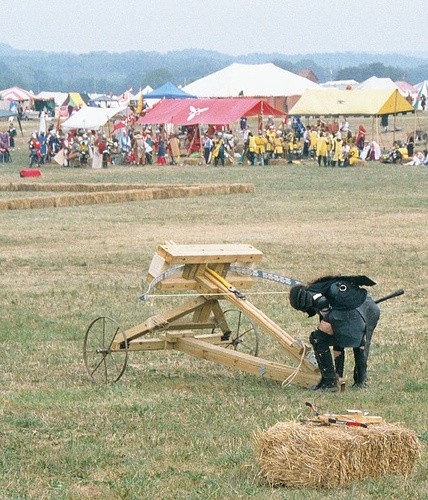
316, 80, 360, 89
392, 80, 412, 91
62, 92, 99, 112
92, 95, 117, 104
0, 86, 36, 101
355, 76, 407, 99
135, 98, 282, 166
407, 80, 427, 111
115, 90, 134, 103
132, 85, 156, 100
34, 90, 70, 108
59, 108, 129, 131
143, 81, 197, 101
182, 61, 322, 98
285, 87, 414, 156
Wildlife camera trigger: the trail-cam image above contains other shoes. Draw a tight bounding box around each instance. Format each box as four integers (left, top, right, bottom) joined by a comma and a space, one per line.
352, 382, 368, 390
314, 377, 339, 392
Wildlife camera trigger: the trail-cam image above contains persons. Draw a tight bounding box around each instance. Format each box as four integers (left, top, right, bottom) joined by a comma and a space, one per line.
1, 114, 427, 168
290, 283, 379, 392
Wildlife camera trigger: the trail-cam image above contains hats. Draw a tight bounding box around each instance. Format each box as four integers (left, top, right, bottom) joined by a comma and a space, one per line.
289, 284, 307, 310
